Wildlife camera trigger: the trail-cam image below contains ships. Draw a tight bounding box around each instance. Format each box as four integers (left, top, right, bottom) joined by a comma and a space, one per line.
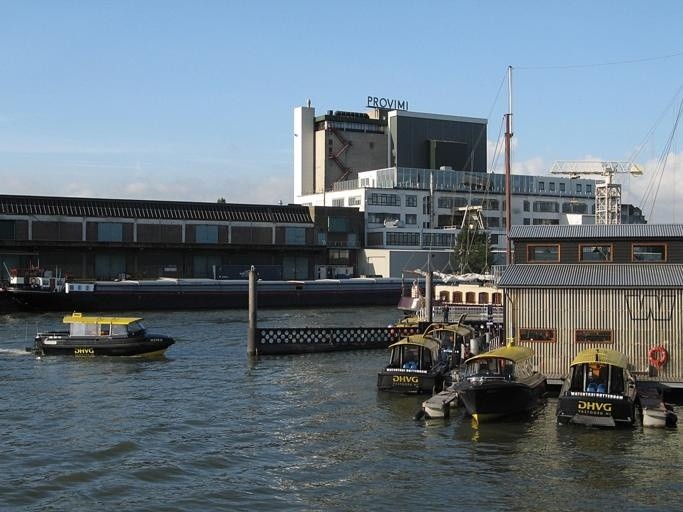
397, 269, 505, 326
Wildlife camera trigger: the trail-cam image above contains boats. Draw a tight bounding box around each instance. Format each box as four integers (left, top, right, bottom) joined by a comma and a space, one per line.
375, 320, 642, 430
26, 311, 176, 359
